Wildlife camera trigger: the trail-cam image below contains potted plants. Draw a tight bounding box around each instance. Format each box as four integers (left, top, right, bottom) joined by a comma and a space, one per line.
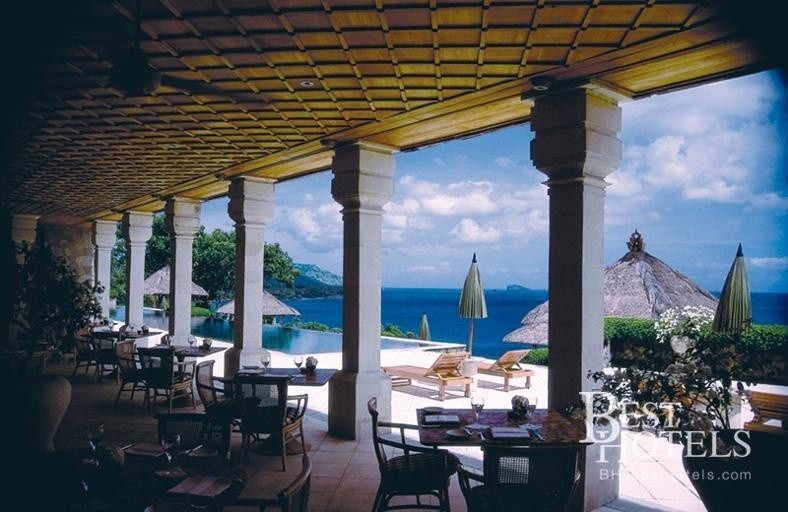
0, 226, 106, 453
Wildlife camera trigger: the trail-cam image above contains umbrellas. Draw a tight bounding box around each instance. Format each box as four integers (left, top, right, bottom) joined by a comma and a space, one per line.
418, 312, 431, 341
458, 252, 487, 357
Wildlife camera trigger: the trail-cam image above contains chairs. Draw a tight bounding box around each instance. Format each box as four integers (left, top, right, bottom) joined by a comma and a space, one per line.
475, 350, 533, 393
383, 350, 474, 401
0, 321, 338, 512
367, 396, 452, 511
455, 440, 595, 511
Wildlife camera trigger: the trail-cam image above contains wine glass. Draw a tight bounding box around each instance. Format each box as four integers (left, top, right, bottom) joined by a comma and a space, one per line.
186, 334, 196, 351
465, 396, 488, 430
520, 398, 540, 429
160, 433, 182, 480
258, 352, 272, 372
293, 355, 307, 379
83, 420, 105, 470
128, 322, 136, 336
107, 320, 114, 332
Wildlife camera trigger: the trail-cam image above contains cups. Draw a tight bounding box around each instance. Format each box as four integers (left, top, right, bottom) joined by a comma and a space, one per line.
136, 325, 143, 336
204, 339, 212, 351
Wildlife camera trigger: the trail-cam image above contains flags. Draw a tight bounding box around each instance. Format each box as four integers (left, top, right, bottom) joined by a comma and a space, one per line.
711, 243, 752, 388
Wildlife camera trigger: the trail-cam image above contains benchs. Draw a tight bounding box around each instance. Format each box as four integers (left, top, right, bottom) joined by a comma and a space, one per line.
744, 391, 788, 434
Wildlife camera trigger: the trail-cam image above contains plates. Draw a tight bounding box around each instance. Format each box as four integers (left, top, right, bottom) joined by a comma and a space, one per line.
448, 429, 474, 440
423, 406, 444, 414
243, 365, 259, 369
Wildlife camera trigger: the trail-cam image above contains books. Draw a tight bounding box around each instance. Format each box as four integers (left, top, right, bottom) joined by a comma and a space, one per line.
424, 413, 460, 423
490, 427, 530, 439
